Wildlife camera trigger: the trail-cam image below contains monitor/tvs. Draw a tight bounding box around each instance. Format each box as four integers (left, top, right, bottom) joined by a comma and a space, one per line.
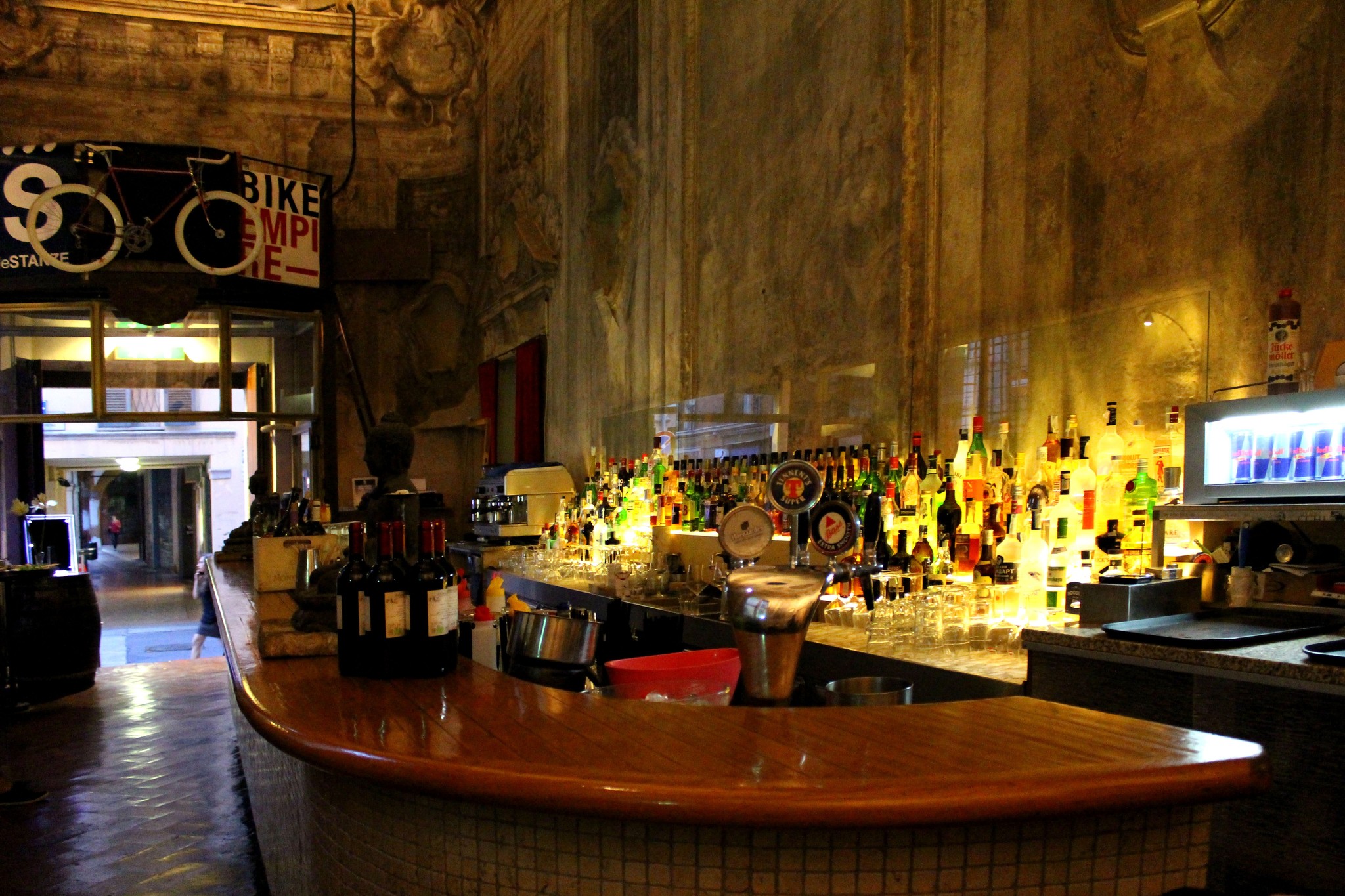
351, 476, 379, 510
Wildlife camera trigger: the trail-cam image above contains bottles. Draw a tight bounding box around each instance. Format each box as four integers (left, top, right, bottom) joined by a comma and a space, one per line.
487, 575, 506, 612
538, 401, 1204, 630
470, 605, 499, 671
334, 518, 463, 678
1265, 287, 1301, 394
251, 487, 333, 537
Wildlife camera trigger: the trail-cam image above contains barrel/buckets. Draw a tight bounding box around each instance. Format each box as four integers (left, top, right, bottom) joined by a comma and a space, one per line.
504, 609, 596, 665
827, 678, 912, 706
604, 647, 740, 705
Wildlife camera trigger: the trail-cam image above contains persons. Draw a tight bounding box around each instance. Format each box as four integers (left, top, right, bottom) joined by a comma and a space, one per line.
193, 555, 221, 658
249, 470, 271, 517
359, 424, 418, 521
109, 516, 121, 549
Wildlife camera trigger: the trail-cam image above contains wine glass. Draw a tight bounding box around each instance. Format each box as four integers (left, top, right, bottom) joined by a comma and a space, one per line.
498, 546, 731, 624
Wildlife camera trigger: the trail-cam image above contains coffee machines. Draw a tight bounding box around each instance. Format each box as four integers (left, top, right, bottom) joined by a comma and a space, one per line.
470, 462, 576, 546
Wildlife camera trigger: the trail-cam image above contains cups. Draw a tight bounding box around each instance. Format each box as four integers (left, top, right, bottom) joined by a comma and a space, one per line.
822, 579, 1034, 663
1293, 304, 1331, 393
295, 547, 322, 590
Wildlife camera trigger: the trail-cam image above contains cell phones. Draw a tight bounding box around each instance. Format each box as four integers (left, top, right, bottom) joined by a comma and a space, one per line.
198, 571, 205, 575
